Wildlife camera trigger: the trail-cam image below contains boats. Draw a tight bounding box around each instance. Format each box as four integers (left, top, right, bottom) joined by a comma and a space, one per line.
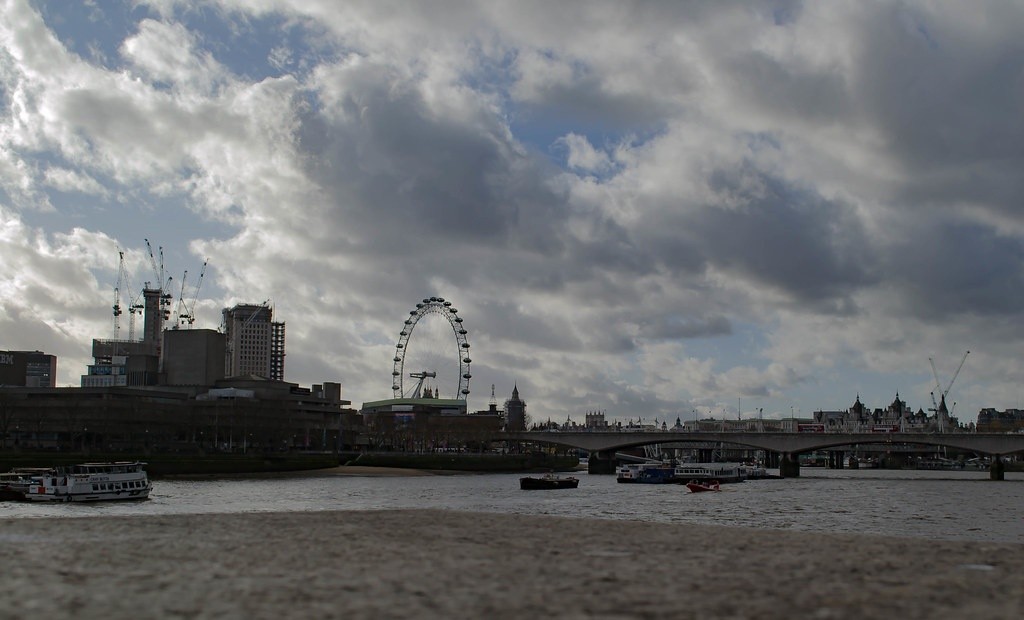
686, 479, 722, 493
674, 462, 744, 485
25, 461, 153, 502
747, 465, 785, 479
615, 457, 684, 484
0, 468, 59, 493
519, 473, 579, 490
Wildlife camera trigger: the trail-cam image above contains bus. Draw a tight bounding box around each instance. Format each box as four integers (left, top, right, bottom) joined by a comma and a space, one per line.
872, 424, 899, 432
798, 423, 825, 433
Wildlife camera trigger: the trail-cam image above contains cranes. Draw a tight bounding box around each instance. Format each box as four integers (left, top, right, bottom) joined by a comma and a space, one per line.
928, 350, 970, 410
112, 238, 210, 340
